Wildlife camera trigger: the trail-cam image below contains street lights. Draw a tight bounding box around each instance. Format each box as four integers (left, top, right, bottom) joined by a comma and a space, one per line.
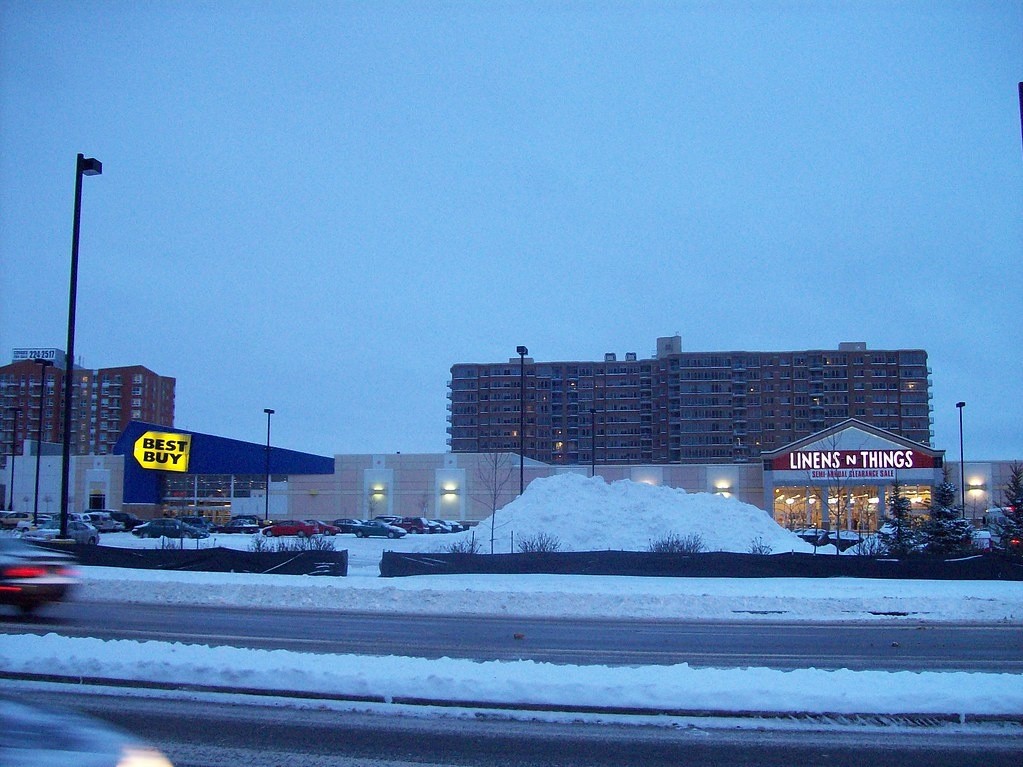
264, 408, 275, 518
589, 409, 596, 476
8, 407, 22, 511
956, 401, 966, 518
61, 152, 100, 532
516, 345, 528, 495
34, 360, 54, 525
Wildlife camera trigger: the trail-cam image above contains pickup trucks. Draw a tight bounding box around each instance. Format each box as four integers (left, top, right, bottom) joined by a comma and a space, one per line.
969, 530, 993, 554
85, 509, 149, 530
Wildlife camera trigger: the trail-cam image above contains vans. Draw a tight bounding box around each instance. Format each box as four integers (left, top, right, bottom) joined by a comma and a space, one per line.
87, 512, 112, 532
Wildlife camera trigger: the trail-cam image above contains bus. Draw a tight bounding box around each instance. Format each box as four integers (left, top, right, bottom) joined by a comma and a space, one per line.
986, 507, 1019, 554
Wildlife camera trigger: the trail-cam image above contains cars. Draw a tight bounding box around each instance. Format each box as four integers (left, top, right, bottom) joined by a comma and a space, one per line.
216, 518, 260, 535
369, 515, 430, 534
132, 517, 210, 540
305, 520, 341, 536
262, 519, 320, 538
428, 519, 464, 533
792, 529, 864, 552
0, 511, 83, 531
0, 539, 80, 616
876, 520, 912, 548
112, 519, 125, 532
17, 517, 52, 533
231, 515, 264, 527
20, 519, 101, 546
209, 522, 219, 532
353, 521, 407, 539
174, 515, 209, 531
332, 518, 369, 533
